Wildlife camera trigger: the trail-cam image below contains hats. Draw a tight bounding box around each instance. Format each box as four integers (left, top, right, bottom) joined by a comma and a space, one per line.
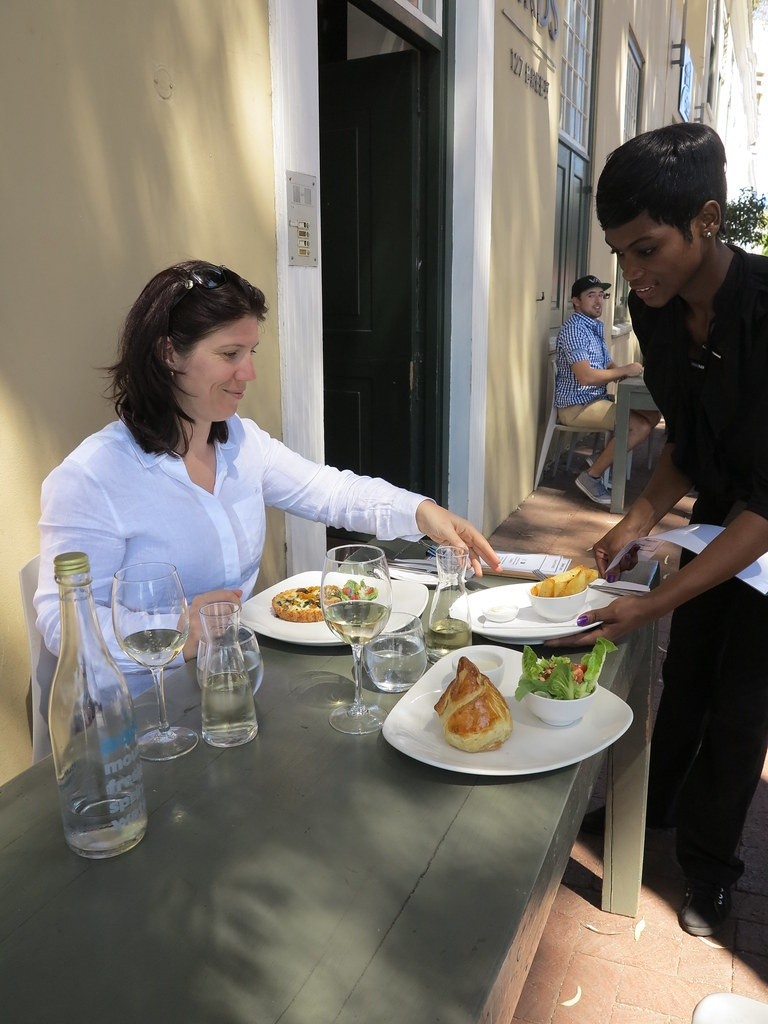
571, 275, 611, 298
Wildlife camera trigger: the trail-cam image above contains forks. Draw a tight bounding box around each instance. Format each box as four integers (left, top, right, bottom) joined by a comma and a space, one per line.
532, 568, 549, 582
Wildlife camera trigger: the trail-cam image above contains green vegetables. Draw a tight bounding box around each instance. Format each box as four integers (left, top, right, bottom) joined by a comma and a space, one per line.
339, 579, 379, 602
514, 635, 618, 702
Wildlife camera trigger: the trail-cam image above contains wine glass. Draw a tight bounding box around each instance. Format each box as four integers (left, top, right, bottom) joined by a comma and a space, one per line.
109, 562, 199, 761
320, 543, 393, 736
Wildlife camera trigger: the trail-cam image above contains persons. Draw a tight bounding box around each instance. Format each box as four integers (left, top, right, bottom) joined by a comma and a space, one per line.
555, 276, 660, 505
544, 123, 768, 936
29, 260, 502, 763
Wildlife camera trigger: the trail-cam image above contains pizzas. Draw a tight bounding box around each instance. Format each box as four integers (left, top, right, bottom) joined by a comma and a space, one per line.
272, 585, 342, 623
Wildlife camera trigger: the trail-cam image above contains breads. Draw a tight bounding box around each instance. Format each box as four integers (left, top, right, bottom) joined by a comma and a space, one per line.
434, 656, 513, 753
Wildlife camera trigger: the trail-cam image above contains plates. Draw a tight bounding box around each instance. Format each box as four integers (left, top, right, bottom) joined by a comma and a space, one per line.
235, 570, 430, 648
382, 645, 634, 777
449, 578, 618, 646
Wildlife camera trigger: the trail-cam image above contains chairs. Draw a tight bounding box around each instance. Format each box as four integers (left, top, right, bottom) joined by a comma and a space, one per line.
533, 359, 653, 492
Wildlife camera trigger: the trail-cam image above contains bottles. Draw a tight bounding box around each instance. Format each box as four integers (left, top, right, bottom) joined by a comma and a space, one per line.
46, 550, 147, 859
198, 600, 259, 749
425, 547, 472, 664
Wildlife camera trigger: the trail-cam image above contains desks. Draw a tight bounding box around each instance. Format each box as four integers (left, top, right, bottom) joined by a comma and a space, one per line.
0, 537, 659, 1023
611, 378, 659, 513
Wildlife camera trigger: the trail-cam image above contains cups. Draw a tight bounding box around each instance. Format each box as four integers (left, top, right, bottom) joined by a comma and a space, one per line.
361, 612, 427, 693
196, 625, 265, 696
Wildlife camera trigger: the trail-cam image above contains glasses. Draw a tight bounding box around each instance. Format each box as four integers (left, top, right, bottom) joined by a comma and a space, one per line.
169, 262, 241, 314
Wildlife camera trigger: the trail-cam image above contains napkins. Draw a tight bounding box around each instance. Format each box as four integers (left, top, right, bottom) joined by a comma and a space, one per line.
388, 566, 468, 585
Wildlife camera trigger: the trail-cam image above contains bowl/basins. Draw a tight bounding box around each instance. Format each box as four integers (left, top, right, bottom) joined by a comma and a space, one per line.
451, 648, 506, 689
480, 599, 520, 623
518, 673, 599, 727
527, 581, 588, 623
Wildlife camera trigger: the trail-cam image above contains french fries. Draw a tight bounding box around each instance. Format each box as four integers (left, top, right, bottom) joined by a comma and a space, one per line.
532, 565, 599, 597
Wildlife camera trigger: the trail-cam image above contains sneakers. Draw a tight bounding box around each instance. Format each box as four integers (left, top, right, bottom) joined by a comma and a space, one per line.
577, 804, 667, 838
680, 876, 733, 938
574, 471, 612, 505
586, 453, 615, 489
